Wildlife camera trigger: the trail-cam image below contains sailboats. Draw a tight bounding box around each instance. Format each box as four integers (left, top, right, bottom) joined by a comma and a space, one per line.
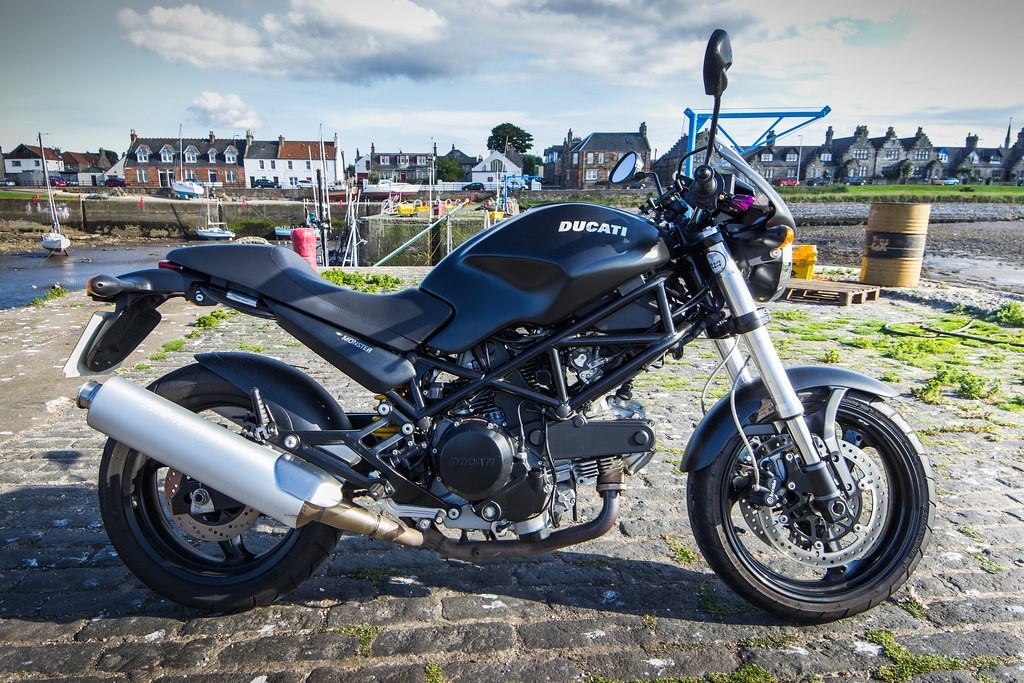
195, 180, 236, 240
273, 121, 367, 266
169, 124, 204, 198
36, 132, 70, 254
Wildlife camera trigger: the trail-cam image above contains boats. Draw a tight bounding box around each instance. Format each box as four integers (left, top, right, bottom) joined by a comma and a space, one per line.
363, 178, 422, 200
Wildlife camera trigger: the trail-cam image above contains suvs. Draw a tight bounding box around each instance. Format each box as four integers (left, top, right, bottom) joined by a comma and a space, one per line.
297, 179, 318, 189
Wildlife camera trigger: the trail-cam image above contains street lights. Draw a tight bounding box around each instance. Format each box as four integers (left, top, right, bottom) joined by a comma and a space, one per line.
968, 138, 984, 185
797, 135, 802, 181
233, 135, 239, 185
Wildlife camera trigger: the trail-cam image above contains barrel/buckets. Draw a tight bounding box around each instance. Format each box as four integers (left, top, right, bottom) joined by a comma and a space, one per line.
858, 201, 931, 288
791, 245, 817, 280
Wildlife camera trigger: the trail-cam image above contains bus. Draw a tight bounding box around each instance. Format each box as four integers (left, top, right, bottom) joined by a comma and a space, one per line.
501, 175, 541, 188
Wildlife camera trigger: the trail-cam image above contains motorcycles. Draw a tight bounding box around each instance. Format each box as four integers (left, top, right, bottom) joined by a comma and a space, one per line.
60, 29, 937, 622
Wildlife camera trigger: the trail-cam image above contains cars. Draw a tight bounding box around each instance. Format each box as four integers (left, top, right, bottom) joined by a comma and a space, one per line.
620, 184, 646, 189
186, 178, 205, 188
105, 178, 127, 187
0, 178, 15, 186
775, 178, 800, 187
44, 177, 79, 188
499, 181, 528, 191
933, 177, 959, 185
541, 178, 548, 184
462, 183, 485, 191
807, 177, 834, 187
254, 179, 282, 189
843, 177, 867, 186
